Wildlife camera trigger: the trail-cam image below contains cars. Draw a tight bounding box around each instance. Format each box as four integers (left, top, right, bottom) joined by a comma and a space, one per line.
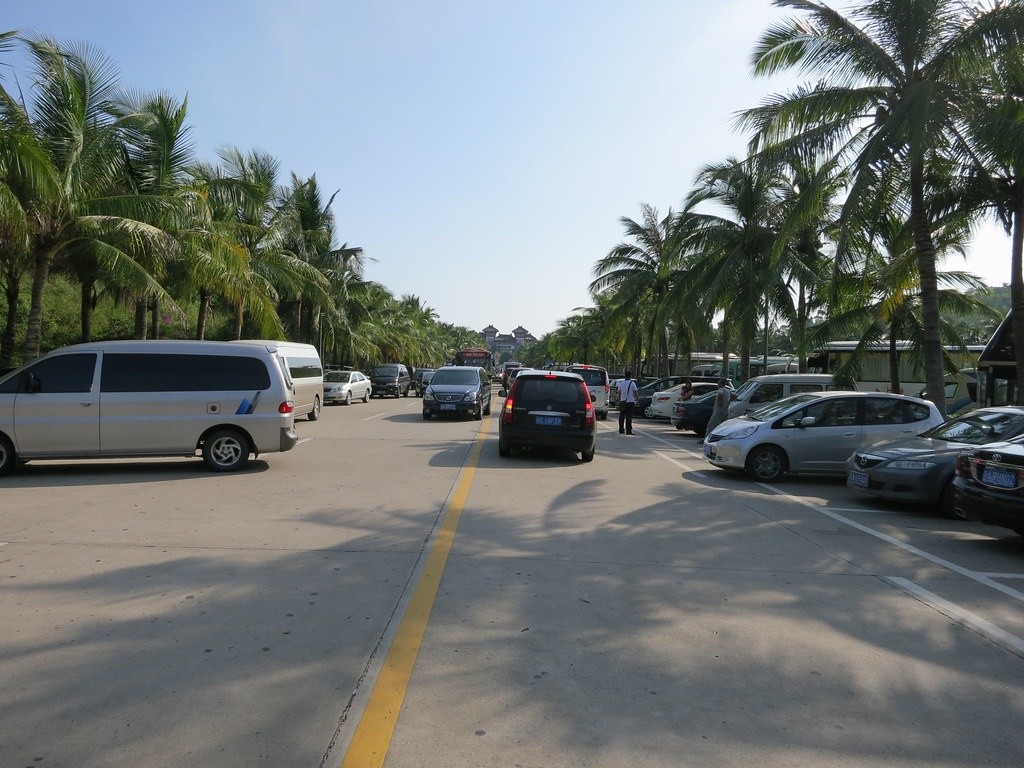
497, 370, 597, 462
411, 368, 437, 398
703, 390, 947, 485
422, 366, 493, 419
950, 431, 1024, 537
323, 370, 372, 405
844, 404, 1024, 518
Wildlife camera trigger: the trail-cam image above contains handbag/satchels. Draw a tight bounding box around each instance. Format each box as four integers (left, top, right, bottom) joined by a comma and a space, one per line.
619, 401, 627, 411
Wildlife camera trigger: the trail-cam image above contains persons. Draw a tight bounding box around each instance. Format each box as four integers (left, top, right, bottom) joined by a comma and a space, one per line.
677, 380, 693, 432
618, 372, 638, 435
705, 379, 730, 438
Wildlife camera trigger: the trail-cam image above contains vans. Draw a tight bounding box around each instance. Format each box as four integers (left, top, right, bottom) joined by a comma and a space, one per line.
727, 373, 860, 430
369, 363, 411, 399
609, 339, 1006, 423
226, 339, 325, 421
1, 338, 299, 472
493, 360, 611, 421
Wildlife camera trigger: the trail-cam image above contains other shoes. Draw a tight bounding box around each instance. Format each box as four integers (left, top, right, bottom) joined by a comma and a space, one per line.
626, 432, 635, 435
697, 440, 704, 444
619, 431, 624, 434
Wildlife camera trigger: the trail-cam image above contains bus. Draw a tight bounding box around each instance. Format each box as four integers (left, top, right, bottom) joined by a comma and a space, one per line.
453, 347, 496, 385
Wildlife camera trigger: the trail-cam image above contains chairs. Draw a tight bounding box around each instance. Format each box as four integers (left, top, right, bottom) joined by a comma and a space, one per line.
820, 405, 846, 426
583, 373, 601, 385
